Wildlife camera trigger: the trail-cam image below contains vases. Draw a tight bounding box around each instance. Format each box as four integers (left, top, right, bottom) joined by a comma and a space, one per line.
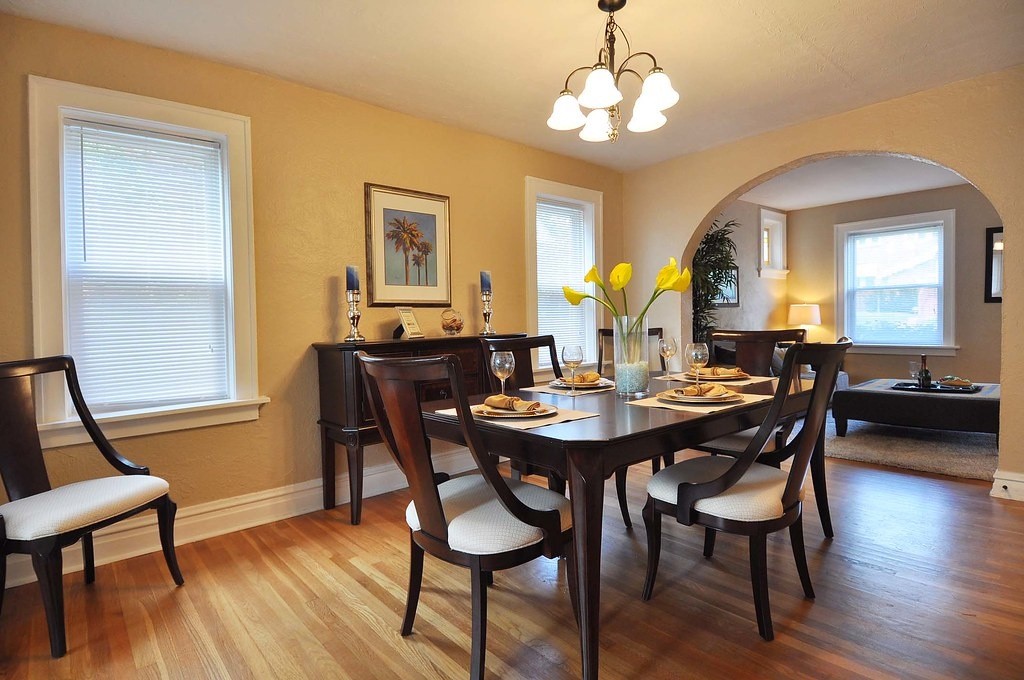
612, 315, 649, 397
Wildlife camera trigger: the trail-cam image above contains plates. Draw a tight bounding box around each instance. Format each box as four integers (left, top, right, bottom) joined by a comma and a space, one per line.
471, 370, 749, 417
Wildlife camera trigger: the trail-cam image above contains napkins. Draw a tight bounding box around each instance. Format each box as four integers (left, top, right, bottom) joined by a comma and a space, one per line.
686, 367, 748, 379
561, 371, 600, 383
481, 394, 539, 411
674, 383, 728, 397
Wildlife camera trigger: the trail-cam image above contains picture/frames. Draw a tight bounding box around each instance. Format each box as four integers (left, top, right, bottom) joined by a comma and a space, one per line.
707, 266, 740, 308
363, 181, 453, 308
396, 307, 425, 339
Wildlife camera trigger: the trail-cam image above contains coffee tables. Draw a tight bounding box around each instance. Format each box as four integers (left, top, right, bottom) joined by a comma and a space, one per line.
832, 378, 1002, 448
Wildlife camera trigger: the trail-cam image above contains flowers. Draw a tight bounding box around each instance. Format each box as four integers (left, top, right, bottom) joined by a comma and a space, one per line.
562, 256, 691, 313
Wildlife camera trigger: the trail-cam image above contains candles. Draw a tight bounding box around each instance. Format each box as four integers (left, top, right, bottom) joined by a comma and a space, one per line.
480, 270, 492, 292
345, 264, 361, 290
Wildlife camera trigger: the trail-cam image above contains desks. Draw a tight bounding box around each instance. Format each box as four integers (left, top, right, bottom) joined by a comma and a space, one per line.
417, 369, 834, 680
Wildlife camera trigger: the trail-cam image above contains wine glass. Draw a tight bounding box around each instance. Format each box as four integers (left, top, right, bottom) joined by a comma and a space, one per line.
490, 351, 515, 395
562, 345, 583, 394
908, 362, 920, 388
684, 343, 709, 385
657, 339, 676, 379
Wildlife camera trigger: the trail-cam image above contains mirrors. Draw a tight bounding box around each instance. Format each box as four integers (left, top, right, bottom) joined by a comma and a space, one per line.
984, 226, 1004, 304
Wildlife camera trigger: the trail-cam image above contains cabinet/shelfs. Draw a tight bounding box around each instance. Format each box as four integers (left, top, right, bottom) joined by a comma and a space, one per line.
311, 332, 529, 520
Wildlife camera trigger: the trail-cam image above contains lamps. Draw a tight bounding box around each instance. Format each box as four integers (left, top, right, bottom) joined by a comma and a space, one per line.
786, 305, 822, 336
546, 0, 678, 143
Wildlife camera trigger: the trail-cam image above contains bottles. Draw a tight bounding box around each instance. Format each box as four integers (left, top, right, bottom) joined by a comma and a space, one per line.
918, 354, 931, 388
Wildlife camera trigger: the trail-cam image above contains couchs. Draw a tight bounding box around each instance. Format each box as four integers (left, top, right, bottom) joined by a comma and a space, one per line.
715, 342, 848, 426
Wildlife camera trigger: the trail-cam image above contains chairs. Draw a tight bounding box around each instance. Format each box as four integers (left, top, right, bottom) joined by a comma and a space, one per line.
354, 350, 583, 679
643, 342, 855, 642
696, 329, 811, 469
0, 354, 185, 659
480, 335, 635, 531
597, 328, 667, 376
685, 337, 849, 537
